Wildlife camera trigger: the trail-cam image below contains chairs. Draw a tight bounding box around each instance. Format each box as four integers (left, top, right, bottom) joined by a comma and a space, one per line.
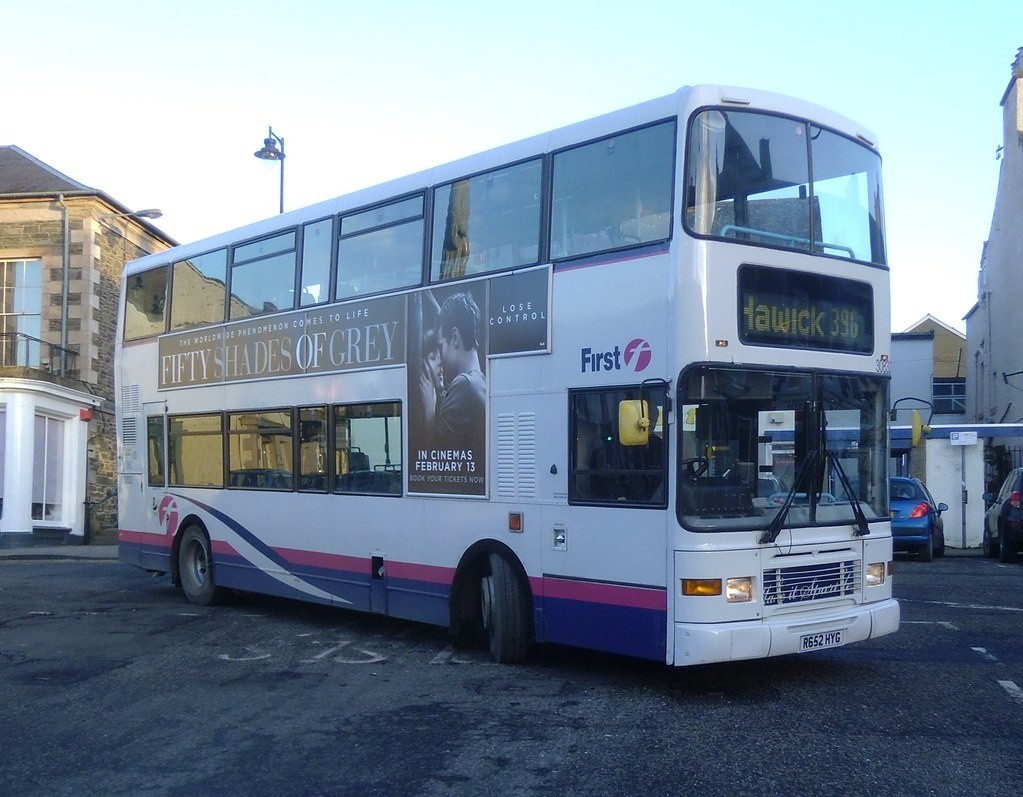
231, 451, 401, 494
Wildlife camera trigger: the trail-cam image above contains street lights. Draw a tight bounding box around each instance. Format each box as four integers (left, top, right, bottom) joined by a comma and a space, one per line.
256, 125, 286, 216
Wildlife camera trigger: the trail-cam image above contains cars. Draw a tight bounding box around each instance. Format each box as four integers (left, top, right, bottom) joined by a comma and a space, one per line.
981, 466, 1023, 559
840, 475, 952, 562
754, 474, 790, 508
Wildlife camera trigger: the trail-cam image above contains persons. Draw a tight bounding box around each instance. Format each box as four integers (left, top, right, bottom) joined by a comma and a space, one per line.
610, 404, 663, 500
421, 290, 486, 445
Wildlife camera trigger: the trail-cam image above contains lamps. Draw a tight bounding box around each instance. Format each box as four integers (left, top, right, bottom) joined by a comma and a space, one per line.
99, 209, 163, 219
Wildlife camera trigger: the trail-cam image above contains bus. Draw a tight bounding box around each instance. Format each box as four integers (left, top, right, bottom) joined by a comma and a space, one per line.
114, 84, 933, 671
